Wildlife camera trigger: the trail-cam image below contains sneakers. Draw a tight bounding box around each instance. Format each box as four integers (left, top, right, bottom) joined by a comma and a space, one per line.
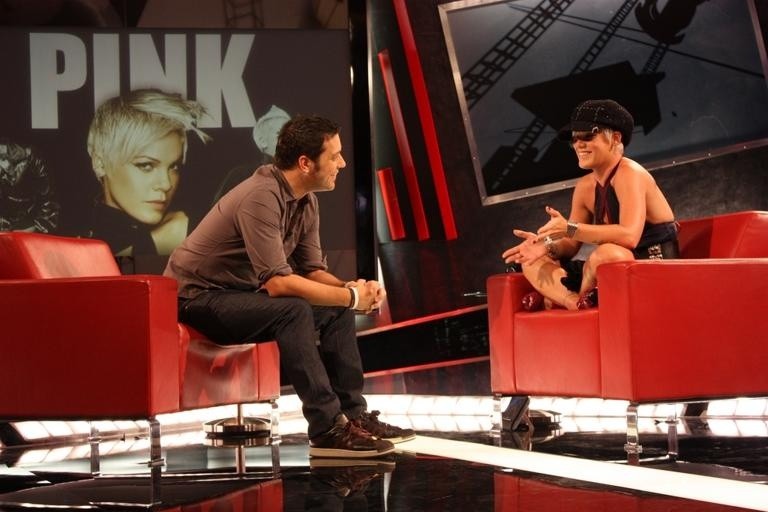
308, 413, 395, 457
349, 412, 417, 444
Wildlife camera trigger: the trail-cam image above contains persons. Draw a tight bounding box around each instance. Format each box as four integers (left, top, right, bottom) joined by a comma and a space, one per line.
69, 89, 207, 276
303, 460, 398, 512
164, 112, 415, 458
503, 97, 678, 310
212, 104, 291, 213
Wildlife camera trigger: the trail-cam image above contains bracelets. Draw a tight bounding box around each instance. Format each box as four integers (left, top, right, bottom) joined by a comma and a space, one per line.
346, 286, 358, 310
566, 221, 577, 239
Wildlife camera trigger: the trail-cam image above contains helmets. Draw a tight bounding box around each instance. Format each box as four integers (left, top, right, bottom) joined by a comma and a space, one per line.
562, 99, 634, 146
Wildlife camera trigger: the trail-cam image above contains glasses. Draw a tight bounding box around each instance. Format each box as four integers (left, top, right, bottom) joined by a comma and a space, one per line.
566, 132, 602, 143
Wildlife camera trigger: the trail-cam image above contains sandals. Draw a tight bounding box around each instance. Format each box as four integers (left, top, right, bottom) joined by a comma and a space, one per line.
577, 283, 598, 311
520, 291, 558, 310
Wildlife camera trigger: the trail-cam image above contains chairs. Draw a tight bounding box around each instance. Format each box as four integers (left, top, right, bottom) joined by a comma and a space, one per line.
486, 210, 768, 464
0, 230, 280, 473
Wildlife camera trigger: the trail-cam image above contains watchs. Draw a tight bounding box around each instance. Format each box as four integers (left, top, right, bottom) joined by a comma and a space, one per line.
542, 235, 562, 260
